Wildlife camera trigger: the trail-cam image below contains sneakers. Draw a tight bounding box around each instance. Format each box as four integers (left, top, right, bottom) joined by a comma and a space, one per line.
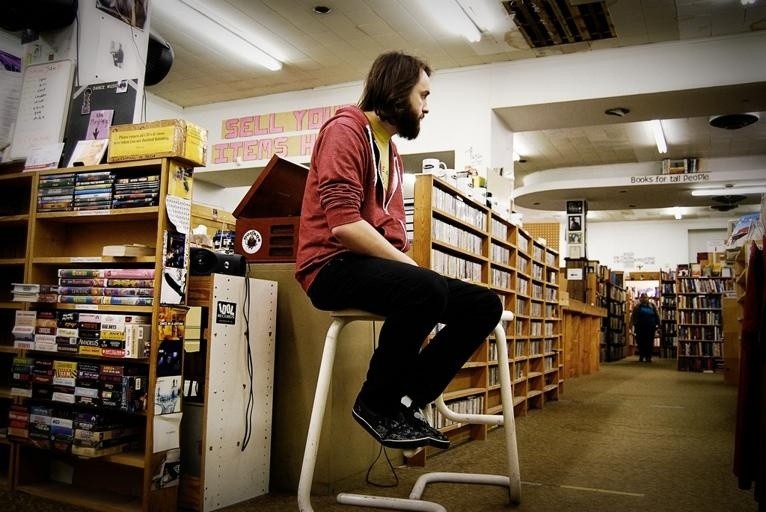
352, 395, 451, 449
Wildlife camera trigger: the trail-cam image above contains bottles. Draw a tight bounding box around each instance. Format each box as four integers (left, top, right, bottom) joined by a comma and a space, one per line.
486, 192, 493, 208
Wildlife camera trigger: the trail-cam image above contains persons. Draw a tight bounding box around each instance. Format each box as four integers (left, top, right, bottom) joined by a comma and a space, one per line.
110, 42, 124, 67
571, 218, 580, 230
295, 52, 502, 448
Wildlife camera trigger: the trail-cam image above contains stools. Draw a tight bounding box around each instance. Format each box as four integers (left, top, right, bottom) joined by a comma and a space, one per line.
292, 297, 527, 511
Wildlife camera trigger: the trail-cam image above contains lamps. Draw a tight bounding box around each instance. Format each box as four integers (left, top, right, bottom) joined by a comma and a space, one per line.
425, 0, 482, 48
150, 0, 285, 76
652, 118, 669, 154
687, 184, 766, 201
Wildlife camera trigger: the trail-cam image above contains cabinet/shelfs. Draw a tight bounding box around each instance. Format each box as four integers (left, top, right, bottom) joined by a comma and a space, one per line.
181, 273, 277, 511
399, 171, 563, 463
565, 262, 631, 372
658, 235, 762, 488
2, 161, 193, 510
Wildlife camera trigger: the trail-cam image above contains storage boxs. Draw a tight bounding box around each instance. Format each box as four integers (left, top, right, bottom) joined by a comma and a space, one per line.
189, 202, 236, 244
231, 157, 310, 262
106, 116, 209, 170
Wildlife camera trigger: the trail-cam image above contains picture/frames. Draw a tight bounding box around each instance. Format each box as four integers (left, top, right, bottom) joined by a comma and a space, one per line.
566, 201, 583, 258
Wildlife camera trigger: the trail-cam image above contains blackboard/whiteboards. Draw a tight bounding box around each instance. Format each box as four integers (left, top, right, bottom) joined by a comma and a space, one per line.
10, 57, 75, 160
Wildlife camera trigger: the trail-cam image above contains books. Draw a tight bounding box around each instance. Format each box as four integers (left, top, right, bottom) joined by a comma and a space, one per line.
401, 184, 557, 432
595, 261, 735, 374
0, 266, 156, 459
35, 171, 162, 213
102, 243, 156, 257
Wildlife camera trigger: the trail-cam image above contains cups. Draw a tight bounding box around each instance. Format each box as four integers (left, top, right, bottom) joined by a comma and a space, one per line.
422, 158, 447, 178
446, 168, 457, 186
